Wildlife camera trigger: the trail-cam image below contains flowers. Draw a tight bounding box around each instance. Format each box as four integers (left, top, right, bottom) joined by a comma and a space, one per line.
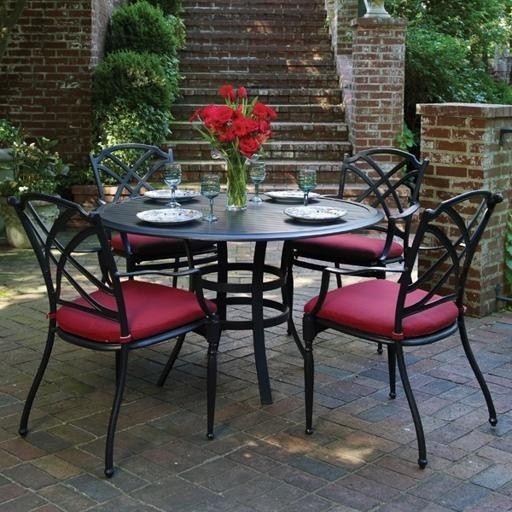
188, 83, 278, 209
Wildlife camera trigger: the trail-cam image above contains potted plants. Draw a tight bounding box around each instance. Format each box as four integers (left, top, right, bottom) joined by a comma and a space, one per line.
6, 137, 74, 250
96, 136, 146, 201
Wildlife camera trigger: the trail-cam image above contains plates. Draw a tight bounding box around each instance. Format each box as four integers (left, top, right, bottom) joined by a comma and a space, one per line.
135, 208, 203, 224
283, 205, 347, 224
266, 190, 321, 203
144, 190, 202, 202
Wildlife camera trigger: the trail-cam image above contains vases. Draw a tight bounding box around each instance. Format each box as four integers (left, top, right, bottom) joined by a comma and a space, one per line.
227, 158, 248, 211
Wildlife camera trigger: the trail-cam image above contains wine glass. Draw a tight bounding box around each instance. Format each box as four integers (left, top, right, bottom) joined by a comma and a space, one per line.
298, 167, 317, 204
164, 162, 183, 207
200, 174, 220, 222
250, 163, 266, 203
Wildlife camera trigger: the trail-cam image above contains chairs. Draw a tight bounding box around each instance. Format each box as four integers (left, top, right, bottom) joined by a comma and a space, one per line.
87, 142, 221, 288
280, 147, 429, 337
7, 191, 227, 479
302, 189, 503, 470
